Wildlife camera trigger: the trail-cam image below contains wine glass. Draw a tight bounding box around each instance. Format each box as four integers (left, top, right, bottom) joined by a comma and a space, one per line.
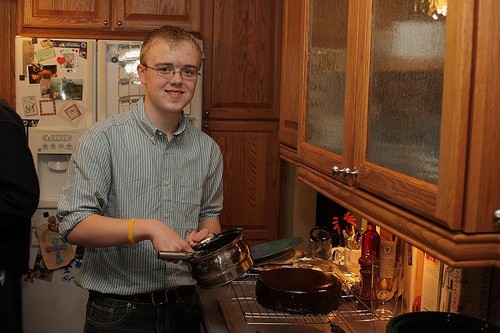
371, 259, 405, 321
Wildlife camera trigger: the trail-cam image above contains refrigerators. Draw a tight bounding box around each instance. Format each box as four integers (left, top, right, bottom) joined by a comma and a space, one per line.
13, 36, 203, 333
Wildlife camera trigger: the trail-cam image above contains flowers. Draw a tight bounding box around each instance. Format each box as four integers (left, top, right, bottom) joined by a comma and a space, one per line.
325, 210, 357, 247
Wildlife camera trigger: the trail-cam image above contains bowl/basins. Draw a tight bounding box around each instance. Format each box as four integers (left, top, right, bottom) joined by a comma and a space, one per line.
388, 310, 499, 332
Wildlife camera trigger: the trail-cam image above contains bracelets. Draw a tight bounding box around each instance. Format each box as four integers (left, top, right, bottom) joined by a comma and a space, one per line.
127, 218, 137, 247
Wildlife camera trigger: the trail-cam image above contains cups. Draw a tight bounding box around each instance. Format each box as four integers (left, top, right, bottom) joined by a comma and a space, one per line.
333, 246, 346, 268
344, 248, 362, 272
308, 227, 333, 262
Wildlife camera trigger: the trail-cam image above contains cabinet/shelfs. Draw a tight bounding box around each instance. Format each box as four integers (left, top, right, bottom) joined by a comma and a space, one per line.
16, 0, 202, 42
295, 0, 500, 269
277, 0, 303, 167
200, 0, 286, 247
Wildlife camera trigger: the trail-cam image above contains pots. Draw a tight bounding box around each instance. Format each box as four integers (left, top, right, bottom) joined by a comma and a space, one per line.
255, 268, 354, 315
158, 228, 254, 289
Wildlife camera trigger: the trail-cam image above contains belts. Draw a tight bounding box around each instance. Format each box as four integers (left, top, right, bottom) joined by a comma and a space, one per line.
89, 285, 195, 306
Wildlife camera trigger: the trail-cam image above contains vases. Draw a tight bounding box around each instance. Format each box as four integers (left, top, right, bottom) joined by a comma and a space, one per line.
336, 244, 345, 265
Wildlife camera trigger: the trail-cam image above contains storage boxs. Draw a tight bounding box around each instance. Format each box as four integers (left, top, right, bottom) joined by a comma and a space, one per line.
402, 242, 424, 313
449, 267, 491, 323
440, 262, 454, 313
420, 252, 444, 313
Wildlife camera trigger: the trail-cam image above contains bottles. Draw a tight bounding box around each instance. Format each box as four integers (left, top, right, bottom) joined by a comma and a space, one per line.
357, 224, 380, 311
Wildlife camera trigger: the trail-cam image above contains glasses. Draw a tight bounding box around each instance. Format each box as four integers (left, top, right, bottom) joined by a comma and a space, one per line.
142, 64, 202, 80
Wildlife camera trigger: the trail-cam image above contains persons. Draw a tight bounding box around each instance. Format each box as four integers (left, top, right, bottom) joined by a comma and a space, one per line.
58, 27, 223, 333
0, 101, 41, 333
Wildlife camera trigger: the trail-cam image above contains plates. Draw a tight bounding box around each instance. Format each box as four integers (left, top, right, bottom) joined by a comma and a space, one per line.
250, 236, 305, 263
